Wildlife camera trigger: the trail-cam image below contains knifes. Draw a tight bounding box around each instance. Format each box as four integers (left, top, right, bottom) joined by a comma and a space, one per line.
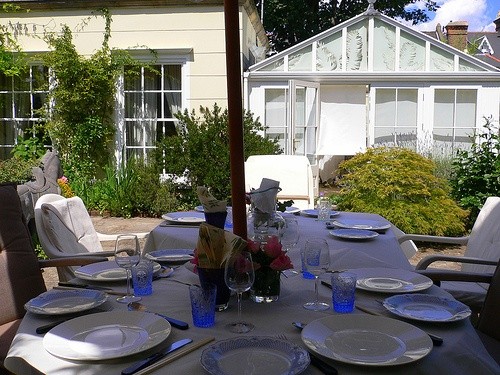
58, 282, 111, 289
120, 338, 193, 375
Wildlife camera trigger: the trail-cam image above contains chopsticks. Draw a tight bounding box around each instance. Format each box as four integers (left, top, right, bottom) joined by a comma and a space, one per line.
132, 336, 215, 375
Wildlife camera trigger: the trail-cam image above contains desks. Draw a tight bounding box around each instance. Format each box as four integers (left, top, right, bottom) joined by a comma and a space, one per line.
142, 211, 419, 270
3, 261, 500, 375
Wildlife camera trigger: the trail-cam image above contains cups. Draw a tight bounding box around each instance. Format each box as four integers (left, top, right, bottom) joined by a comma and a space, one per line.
332, 271, 356, 313
224, 207, 234, 232
317, 198, 331, 224
189, 286, 217, 328
130, 259, 154, 297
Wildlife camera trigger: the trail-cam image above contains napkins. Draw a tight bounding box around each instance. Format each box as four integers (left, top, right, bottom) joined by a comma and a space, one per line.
197, 186, 226, 213
251, 178, 280, 213
196, 223, 248, 267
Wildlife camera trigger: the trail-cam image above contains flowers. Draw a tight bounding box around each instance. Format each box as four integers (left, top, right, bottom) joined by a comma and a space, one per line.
245, 188, 294, 230
189, 235, 293, 297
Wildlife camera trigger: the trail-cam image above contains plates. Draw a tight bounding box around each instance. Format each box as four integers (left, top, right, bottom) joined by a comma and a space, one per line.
44, 311, 172, 361
340, 267, 434, 292
74, 259, 161, 281
162, 211, 205, 224
199, 334, 311, 375
194, 206, 203, 211
332, 217, 391, 230
145, 248, 195, 262
300, 313, 432, 366
303, 210, 339, 217
329, 229, 379, 239
382, 293, 471, 323
277, 207, 300, 213
24, 289, 108, 315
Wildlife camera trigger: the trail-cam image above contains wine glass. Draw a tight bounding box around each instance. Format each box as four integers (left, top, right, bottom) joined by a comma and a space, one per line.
303, 238, 331, 312
114, 234, 144, 304
223, 251, 255, 333
279, 219, 300, 277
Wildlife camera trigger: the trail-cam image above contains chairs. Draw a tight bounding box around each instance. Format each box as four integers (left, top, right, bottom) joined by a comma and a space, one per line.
398, 196, 500, 309
474, 258, 500, 366
244, 154, 315, 210
0, 182, 108, 375
35, 194, 150, 283
